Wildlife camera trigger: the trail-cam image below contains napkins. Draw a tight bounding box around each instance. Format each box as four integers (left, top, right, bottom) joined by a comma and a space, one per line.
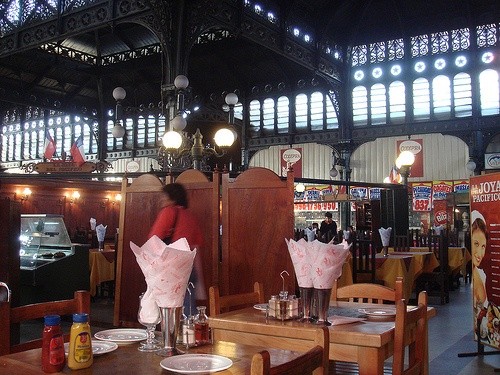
307, 236, 353, 289
285, 237, 335, 288
130, 235, 197, 308
89, 218, 107, 241
378, 227, 392, 246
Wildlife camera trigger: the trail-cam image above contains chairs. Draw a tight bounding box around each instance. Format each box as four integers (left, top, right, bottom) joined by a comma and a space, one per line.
0, 290, 91, 355
208, 281, 264, 315
251, 327, 329, 375
294, 228, 472, 304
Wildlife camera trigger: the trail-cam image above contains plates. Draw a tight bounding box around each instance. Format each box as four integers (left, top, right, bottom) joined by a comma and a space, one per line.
94, 329, 148, 346
358, 307, 397, 318
253, 304, 270, 312
64, 340, 118, 357
159, 354, 233, 374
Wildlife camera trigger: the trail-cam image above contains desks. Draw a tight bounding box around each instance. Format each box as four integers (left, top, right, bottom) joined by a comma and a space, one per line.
89, 242, 115, 303
208, 300, 437, 375
381, 246, 467, 281
391, 252, 439, 281
355, 253, 414, 302
0, 331, 306, 375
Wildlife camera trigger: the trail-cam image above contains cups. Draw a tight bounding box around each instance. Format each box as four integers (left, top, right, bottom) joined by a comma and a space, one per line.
176, 306, 187, 343
383, 245, 389, 257
299, 285, 333, 325
98, 241, 105, 251
155, 306, 185, 357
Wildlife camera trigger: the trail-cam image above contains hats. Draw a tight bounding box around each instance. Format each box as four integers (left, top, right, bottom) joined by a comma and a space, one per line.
471, 210, 486, 226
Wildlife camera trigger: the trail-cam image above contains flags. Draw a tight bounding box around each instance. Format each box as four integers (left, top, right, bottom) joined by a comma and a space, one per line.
70, 135, 85, 166
45, 130, 55, 160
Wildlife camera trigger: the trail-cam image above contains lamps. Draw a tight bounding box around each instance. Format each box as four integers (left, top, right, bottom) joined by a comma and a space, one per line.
329, 148, 476, 183
110, 75, 238, 157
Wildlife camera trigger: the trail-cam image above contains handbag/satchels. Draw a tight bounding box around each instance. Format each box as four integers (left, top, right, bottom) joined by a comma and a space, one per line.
161, 206, 179, 245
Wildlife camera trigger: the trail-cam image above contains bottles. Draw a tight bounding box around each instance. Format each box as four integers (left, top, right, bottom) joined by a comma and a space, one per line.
67, 313, 93, 370
184, 316, 196, 348
194, 306, 210, 346
269, 292, 301, 320
87, 230, 98, 249
41, 315, 66, 373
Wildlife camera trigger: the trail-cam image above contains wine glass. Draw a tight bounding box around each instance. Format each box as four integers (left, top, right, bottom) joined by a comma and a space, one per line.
138, 291, 164, 353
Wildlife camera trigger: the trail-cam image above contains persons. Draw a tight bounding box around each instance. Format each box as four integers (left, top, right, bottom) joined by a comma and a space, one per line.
149, 183, 200, 316
312, 223, 319, 234
471, 210, 490, 344
320, 212, 337, 238
